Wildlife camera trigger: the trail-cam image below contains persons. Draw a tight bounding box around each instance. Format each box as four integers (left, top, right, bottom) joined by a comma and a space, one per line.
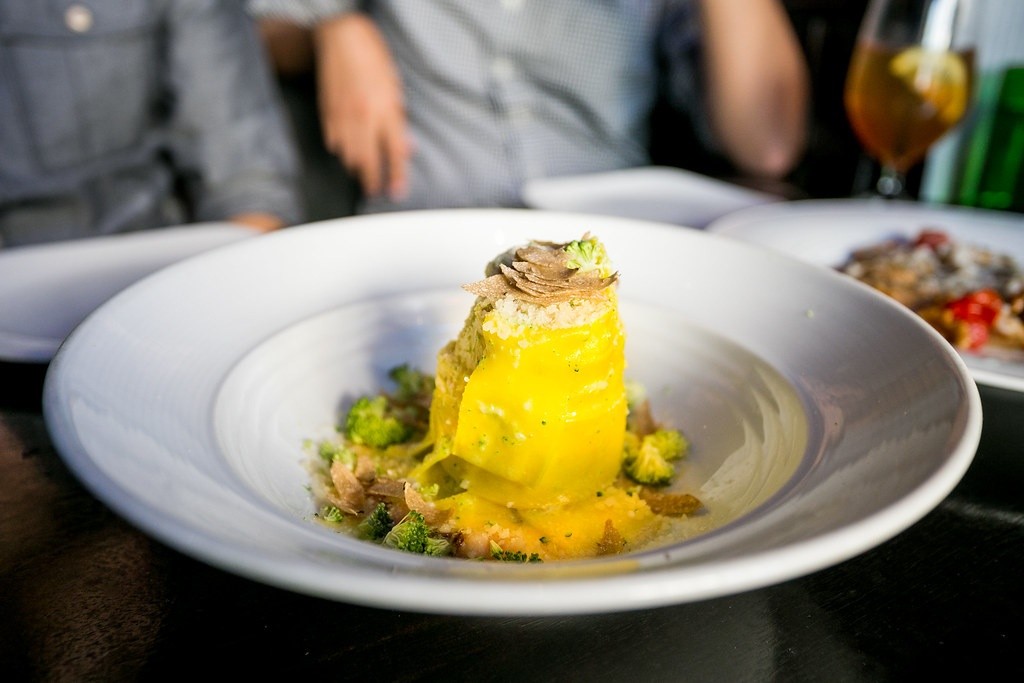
243, 0, 807, 214
0, 0, 302, 249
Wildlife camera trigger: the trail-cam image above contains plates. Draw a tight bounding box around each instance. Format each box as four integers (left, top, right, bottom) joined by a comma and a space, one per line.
533, 165, 776, 225
42, 206, 983, 616
0, 224, 259, 367
706, 203, 1024, 395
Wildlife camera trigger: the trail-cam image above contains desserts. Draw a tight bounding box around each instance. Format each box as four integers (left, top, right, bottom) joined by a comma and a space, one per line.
310, 229, 692, 560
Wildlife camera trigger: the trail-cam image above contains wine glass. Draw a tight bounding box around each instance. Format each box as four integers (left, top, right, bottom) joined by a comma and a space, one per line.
848, 0, 992, 198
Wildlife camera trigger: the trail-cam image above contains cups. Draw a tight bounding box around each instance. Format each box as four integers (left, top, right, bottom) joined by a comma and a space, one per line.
918, 0, 1024, 210
